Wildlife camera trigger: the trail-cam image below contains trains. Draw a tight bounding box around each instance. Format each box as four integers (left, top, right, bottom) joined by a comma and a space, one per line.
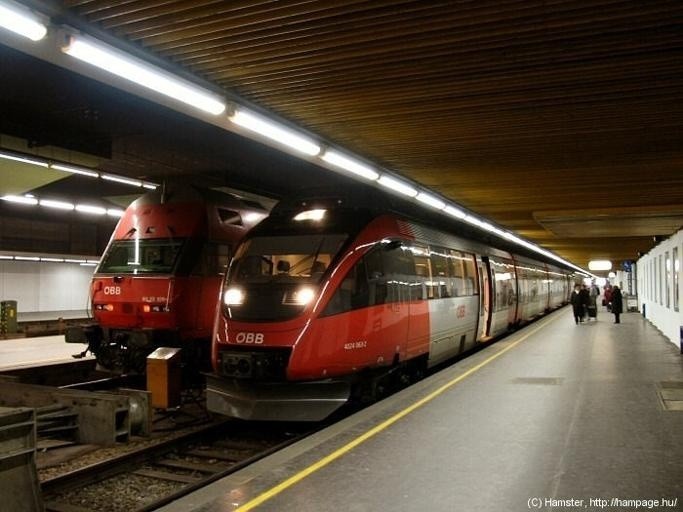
72, 180, 271, 381
201, 186, 582, 426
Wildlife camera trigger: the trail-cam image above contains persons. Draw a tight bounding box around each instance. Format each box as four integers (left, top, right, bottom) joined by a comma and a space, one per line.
571, 281, 623, 325
